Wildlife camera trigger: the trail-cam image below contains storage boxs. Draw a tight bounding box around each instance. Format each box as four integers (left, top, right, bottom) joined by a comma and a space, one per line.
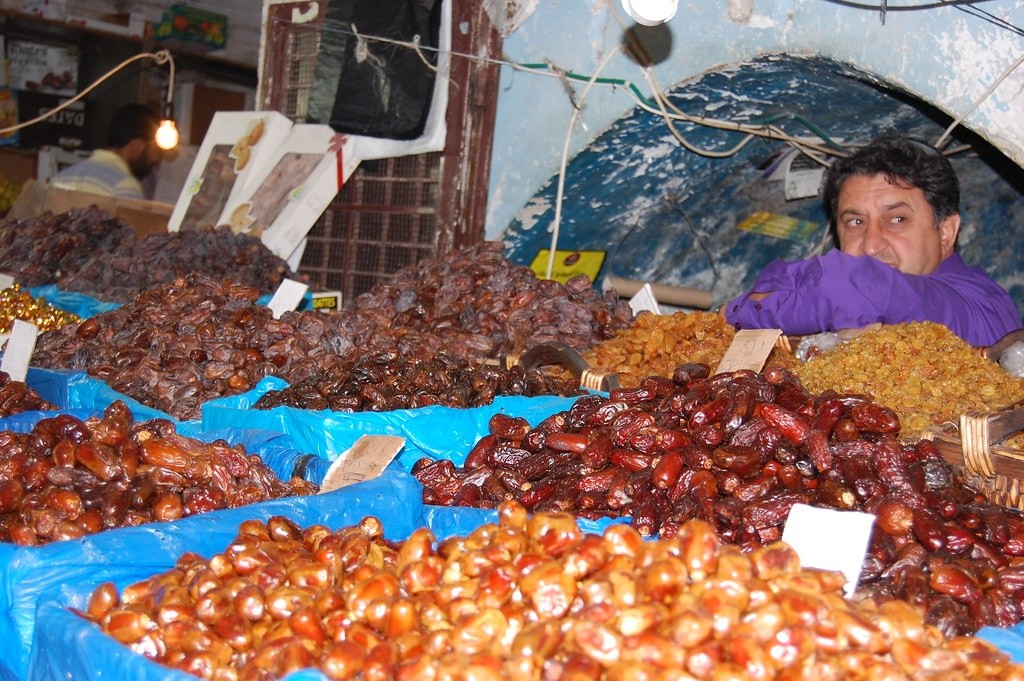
168, 112, 364, 262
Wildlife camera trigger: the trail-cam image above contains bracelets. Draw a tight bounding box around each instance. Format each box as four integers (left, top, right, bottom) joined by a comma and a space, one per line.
712, 304, 725, 314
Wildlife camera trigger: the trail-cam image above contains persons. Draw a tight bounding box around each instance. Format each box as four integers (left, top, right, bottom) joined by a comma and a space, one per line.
711, 135, 1024, 347
46, 103, 161, 200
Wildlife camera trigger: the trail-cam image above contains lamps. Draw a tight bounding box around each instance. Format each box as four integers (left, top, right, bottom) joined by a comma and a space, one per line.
621, 0, 680, 27
